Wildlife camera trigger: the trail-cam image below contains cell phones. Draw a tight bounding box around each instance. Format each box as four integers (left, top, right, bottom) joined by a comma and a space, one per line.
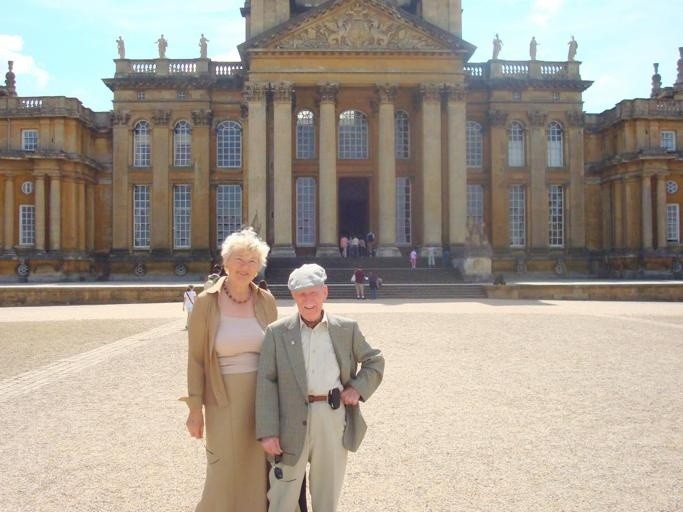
328, 388, 341, 409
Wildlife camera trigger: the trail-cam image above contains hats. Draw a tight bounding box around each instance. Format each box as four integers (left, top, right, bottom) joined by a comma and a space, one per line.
287, 262, 327, 292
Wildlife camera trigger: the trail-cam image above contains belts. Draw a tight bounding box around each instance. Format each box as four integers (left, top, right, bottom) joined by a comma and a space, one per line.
308, 395, 342, 403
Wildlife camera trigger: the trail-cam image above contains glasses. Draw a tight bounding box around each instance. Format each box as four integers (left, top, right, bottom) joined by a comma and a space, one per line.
275, 452, 284, 479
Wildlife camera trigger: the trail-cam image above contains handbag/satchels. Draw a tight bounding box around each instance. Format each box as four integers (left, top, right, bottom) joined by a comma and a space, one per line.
350, 274, 356, 282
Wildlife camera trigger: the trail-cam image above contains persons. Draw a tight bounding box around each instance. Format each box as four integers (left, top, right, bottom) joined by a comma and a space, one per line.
198, 33, 210, 59
178, 227, 277, 512
441, 240, 450, 268
256, 279, 271, 295
181, 284, 197, 332
255, 262, 385, 511
426, 243, 436, 268
338, 229, 383, 299
567, 35, 578, 62
492, 33, 503, 59
529, 36, 541, 61
409, 247, 417, 268
154, 33, 168, 59
201, 263, 221, 291
115, 36, 124, 60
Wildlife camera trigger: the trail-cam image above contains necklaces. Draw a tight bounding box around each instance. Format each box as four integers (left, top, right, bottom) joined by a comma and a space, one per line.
222, 280, 252, 304
